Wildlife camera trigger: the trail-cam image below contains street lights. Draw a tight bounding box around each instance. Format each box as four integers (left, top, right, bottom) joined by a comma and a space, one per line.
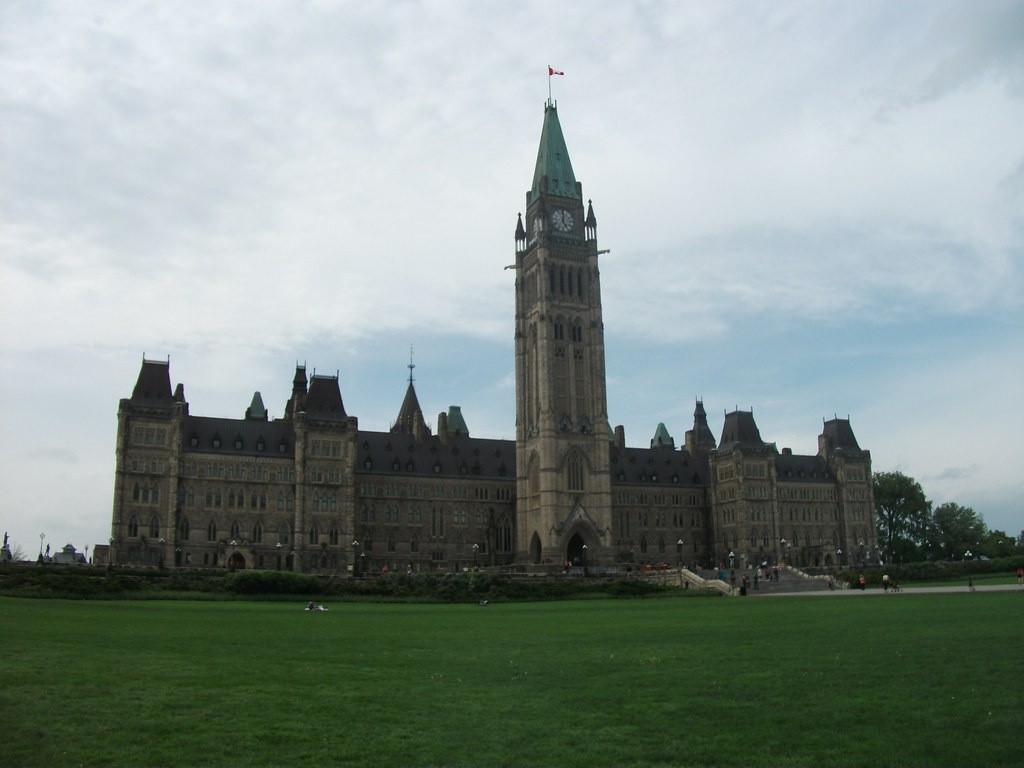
860, 541, 867, 568
473, 543, 479, 573
875, 544, 880, 562
276, 542, 282, 571
83, 544, 89, 562
781, 539, 785, 561
729, 551, 736, 584
36, 532, 45, 566
582, 545, 589, 578
230, 540, 238, 573
352, 540, 359, 574
836, 549, 843, 570
677, 539, 684, 565
964, 550, 974, 586
107, 536, 114, 570
786, 543, 792, 566
158, 538, 166, 570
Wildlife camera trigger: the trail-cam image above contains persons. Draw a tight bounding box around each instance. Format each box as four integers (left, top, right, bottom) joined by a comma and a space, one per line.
646, 555, 780, 597
45, 543, 50, 556
1017, 566, 1023, 583
309, 563, 412, 612
562, 556, 580, 575
829, 574, 902, 593
3, 532, 10, 548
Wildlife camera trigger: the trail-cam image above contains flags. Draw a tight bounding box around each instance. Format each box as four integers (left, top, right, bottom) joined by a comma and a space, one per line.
548, 67, 564, 75
